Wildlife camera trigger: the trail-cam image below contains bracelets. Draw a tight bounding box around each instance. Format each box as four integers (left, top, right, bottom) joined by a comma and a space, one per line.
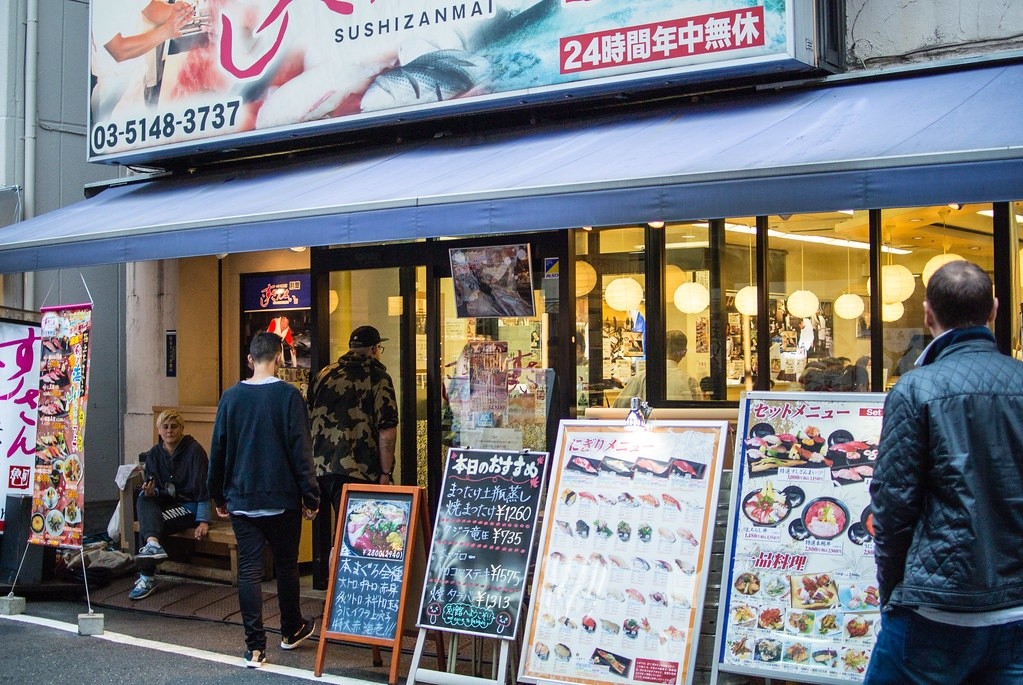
380, 469, 394, 475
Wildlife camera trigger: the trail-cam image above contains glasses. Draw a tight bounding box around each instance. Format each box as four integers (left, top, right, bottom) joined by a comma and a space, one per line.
372, 344, 384, 355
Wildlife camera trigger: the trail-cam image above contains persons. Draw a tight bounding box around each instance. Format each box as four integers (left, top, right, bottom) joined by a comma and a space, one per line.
859, 260, 1023, 685
304, 325, 399, 590
577, 329, 585, 354
725, 323, 734, 362
129, 409, 212, 600
206, 331, 321, 667
788, 356, 872, 392
613, 329, 704, 406
92, 0, 194, 103
798, 316, 815, 352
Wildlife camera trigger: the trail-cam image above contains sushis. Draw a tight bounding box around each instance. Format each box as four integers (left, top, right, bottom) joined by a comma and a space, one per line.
536, 459, 699, 661
745, 433, 879, 482
35, 338, 68, 464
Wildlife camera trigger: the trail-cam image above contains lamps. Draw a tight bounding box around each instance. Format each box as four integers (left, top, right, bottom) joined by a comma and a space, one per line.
575, 210, 966, 322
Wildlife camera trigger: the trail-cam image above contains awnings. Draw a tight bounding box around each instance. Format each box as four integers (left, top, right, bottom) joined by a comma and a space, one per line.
0, 60, 1023, 268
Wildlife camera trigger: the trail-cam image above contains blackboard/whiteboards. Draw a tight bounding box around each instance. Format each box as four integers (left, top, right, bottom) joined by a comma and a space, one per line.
322, 483, 444, 647
415, 447, 550, 640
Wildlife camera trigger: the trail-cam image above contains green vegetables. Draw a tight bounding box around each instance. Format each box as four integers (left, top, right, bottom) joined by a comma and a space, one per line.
364, 510, 399, 534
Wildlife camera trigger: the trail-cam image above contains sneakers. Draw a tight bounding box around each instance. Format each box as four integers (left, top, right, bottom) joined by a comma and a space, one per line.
281, 615, 316, 649
128, 574, 160, 600
243, 648, 268, 668
133, 542, 168, 565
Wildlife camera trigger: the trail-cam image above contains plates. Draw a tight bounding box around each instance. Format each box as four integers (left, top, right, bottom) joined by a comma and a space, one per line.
746, 423, 830, 479
827, 439, 879, 488
566, 455, 706, 481
30, 454, 83, 537
732, 571, 884, 673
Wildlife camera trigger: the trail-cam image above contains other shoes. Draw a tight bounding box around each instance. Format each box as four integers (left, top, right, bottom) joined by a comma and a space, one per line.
312, 578, 328, 591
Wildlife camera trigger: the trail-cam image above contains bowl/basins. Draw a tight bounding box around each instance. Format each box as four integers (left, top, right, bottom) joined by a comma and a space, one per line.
742, 487, 792, 528
861, 503, 876, 540
801, 496, 851, 540
344, 501, 409, 557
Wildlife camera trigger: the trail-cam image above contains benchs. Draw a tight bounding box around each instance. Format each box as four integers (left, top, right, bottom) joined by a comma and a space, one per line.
116, 477, 242, 581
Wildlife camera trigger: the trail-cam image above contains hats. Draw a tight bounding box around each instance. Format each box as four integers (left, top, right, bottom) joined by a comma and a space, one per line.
349, 326, 389, 348
